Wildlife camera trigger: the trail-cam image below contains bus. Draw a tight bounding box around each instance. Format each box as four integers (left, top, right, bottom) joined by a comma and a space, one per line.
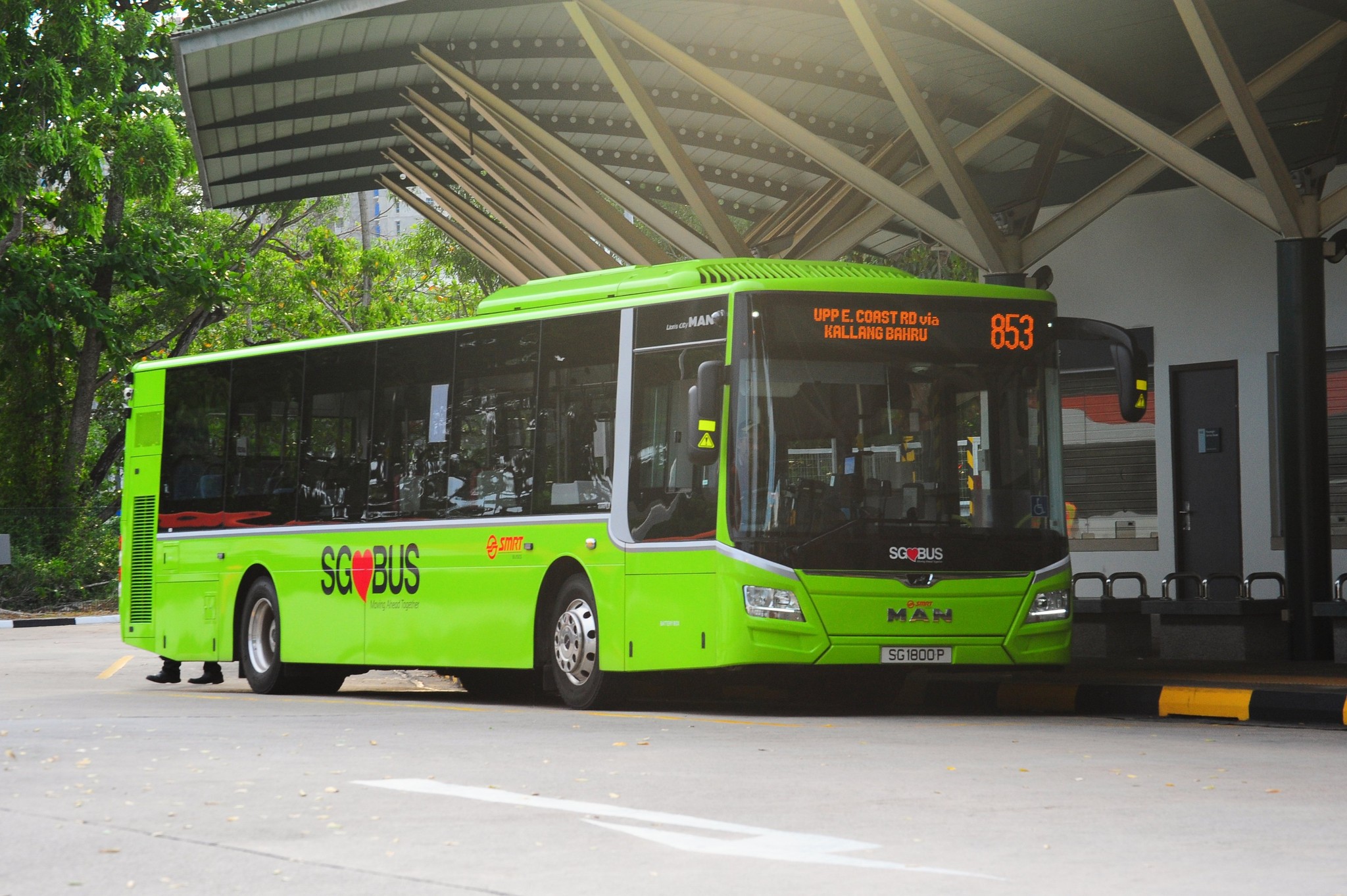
116, 254, 1151, 712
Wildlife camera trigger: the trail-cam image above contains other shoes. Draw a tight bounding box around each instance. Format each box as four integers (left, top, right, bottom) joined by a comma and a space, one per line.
146, 671, 181, 684
188, 671, 224, 684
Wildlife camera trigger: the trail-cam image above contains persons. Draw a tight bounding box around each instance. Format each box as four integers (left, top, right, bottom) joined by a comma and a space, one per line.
146, 655, 223, 685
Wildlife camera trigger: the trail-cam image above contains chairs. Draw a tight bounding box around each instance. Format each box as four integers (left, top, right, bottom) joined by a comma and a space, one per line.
174, 454, 588, 520
